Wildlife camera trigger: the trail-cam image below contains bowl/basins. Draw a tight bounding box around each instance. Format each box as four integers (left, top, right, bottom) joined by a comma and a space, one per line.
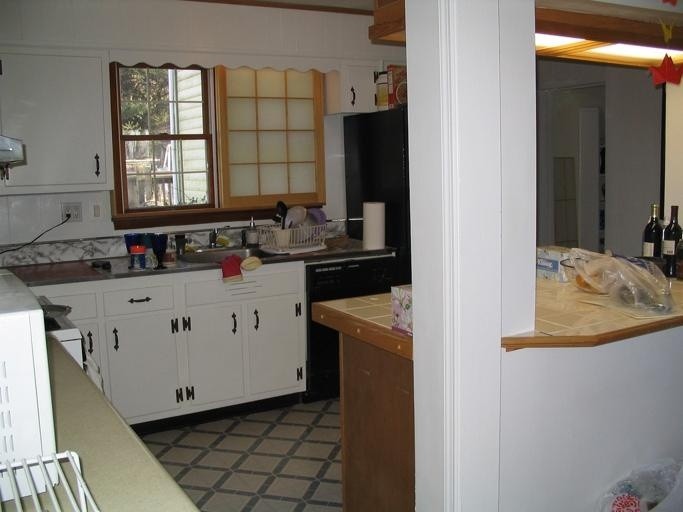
325, 236, 348, 248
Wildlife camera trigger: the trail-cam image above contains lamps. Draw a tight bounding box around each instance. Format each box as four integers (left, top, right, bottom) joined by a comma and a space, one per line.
366, 1, 683, 74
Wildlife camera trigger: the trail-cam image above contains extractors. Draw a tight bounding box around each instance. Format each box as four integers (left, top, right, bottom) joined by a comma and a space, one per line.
0, 137, 26, 168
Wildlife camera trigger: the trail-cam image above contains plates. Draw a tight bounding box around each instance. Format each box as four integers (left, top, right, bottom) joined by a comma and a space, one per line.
284, 206, 338, 243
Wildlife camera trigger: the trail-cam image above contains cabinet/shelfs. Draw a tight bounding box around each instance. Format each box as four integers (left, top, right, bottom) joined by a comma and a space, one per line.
325, 61, 385, 115
5, 48, 114, 196
182, 258, 306, 429
309, 281, 414, 510
26, 271, 185, 427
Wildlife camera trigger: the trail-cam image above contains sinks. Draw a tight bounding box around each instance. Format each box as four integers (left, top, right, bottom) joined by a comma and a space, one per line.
195, 247, 288, 263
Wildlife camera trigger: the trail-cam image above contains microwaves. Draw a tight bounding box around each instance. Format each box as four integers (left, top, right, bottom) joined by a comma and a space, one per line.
0, 264, 58, 505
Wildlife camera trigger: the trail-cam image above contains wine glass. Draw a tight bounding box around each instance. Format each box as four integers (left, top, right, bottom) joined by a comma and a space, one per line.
124, 230, 169, 271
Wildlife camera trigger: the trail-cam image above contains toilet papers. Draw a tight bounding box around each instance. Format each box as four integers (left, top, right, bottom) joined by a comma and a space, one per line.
362, 202, 386, 251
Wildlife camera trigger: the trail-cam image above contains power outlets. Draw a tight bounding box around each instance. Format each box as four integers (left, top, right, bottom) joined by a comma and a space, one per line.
60, 200, 83, 223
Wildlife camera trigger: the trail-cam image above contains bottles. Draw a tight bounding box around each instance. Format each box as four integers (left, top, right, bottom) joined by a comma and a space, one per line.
640, 203, 661, 262
175, 234, 187, 255
244, 217, 259, 248
209, 228, 216, 248
662, 203, 683, 279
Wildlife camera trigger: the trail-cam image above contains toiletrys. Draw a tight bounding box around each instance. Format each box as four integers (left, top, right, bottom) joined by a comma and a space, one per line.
245, 215, 260, 248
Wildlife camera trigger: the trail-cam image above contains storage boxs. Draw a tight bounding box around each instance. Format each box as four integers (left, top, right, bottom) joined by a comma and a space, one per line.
386, 282, 413, 339
536, 245, 574, 283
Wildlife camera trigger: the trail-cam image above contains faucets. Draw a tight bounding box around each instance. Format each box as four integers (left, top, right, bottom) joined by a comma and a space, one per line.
211, 227, 218, 248
